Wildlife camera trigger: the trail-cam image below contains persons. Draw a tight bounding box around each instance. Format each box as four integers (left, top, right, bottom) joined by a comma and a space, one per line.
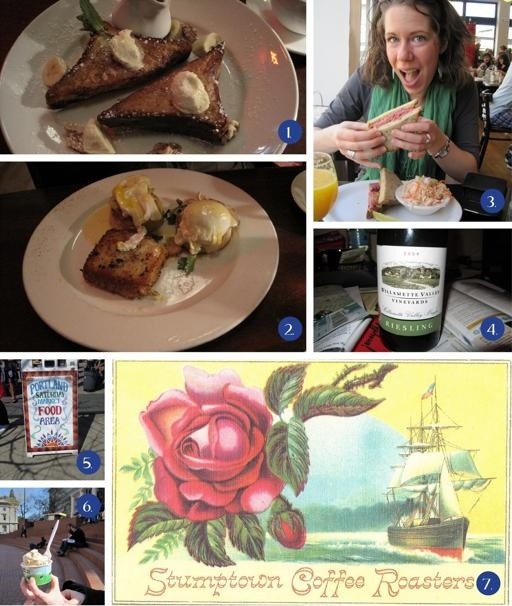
19, 573, 104, 605
0, 360, 19, 436
313, 0, 480, 184
472, 43, 512, 170
20, 523, 86, 557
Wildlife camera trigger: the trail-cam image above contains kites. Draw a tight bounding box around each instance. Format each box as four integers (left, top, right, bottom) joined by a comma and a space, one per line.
314, 277, 512, 351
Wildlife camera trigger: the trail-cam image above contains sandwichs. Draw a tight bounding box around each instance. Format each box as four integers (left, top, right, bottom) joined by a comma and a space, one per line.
366, 167, 403, 218
366, 98, 422, 153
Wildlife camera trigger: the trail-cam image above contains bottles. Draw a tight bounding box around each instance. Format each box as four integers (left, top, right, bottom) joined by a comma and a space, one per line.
374, 231, 450, 352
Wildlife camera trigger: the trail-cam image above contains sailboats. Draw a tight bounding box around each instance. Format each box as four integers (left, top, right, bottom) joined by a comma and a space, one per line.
388, 375, 497, 563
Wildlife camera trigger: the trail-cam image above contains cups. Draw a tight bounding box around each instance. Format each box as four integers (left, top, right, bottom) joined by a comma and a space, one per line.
21, 562, 52, 588
276, 0, 305, 35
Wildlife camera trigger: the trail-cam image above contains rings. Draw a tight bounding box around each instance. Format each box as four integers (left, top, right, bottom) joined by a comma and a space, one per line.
346, 150, 354, 160
424, 133, 431, 144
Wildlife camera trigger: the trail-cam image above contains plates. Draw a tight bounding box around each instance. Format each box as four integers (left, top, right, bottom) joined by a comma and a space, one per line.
246, 0, 305, 54
0, 0, 300, 154
22, 166, 280, 352
322, 178, 462, 224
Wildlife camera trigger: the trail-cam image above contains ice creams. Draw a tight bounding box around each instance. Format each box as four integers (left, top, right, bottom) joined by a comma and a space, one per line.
22, 549, 50, 567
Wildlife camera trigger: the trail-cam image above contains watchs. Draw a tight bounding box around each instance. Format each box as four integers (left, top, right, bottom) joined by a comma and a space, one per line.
428, 134, 450, 159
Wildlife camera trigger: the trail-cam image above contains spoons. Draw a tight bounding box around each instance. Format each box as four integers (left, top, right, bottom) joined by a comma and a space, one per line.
43, 520, 60, 562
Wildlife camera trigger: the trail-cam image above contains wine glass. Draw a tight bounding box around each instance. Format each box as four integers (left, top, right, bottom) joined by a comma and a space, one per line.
311, 153, 338, 224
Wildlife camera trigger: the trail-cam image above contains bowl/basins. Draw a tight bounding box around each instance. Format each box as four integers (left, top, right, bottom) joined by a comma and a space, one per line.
397, 178, 451, 218
